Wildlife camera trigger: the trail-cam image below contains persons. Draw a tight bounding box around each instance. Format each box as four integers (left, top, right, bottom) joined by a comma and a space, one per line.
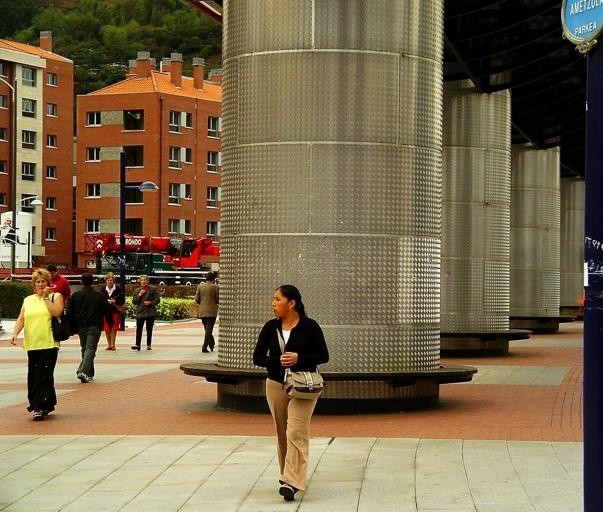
130, 275, 160, 351
45, 265, 70, 315
99, 272, 124, 351
253, 284, 328, 500
9, 267, 63, 420
65, 273, 113, 382
194, 272, 218, 352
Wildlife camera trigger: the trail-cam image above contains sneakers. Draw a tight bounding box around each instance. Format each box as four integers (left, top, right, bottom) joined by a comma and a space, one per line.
147, 347, 151, 350
131, 346, 140, 351
202, 342, 215, 352
279, 484, 294, 502
88, 377, 95, 380
32, 410, 48, 420
77, 371, 89, 383
106, 346, 116, 351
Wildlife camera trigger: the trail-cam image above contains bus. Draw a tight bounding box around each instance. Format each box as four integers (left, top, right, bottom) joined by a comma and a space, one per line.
2, 271, 209, 288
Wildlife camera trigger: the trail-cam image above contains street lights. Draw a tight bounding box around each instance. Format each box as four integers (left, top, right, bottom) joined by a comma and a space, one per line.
116, 151, 159, 331
10, 194, 43, 273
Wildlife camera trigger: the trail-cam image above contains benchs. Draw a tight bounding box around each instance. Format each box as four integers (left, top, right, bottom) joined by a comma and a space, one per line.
560, 304, 584, 309
440, 330, 532, 341
180, 361, 478, 387
509, 315, 577, 322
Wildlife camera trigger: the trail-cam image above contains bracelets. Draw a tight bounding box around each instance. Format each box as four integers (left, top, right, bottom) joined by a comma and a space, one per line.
42, 296, 50, 302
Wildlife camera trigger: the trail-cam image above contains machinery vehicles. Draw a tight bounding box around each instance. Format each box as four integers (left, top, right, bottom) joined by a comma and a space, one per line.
82, 230, 222, 295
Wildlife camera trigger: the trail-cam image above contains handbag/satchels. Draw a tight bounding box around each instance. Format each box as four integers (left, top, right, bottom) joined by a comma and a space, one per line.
117, 304, 128, 311
65, 310, 77, 336
283, 371, 324, 400
51, 313, 70, 342
